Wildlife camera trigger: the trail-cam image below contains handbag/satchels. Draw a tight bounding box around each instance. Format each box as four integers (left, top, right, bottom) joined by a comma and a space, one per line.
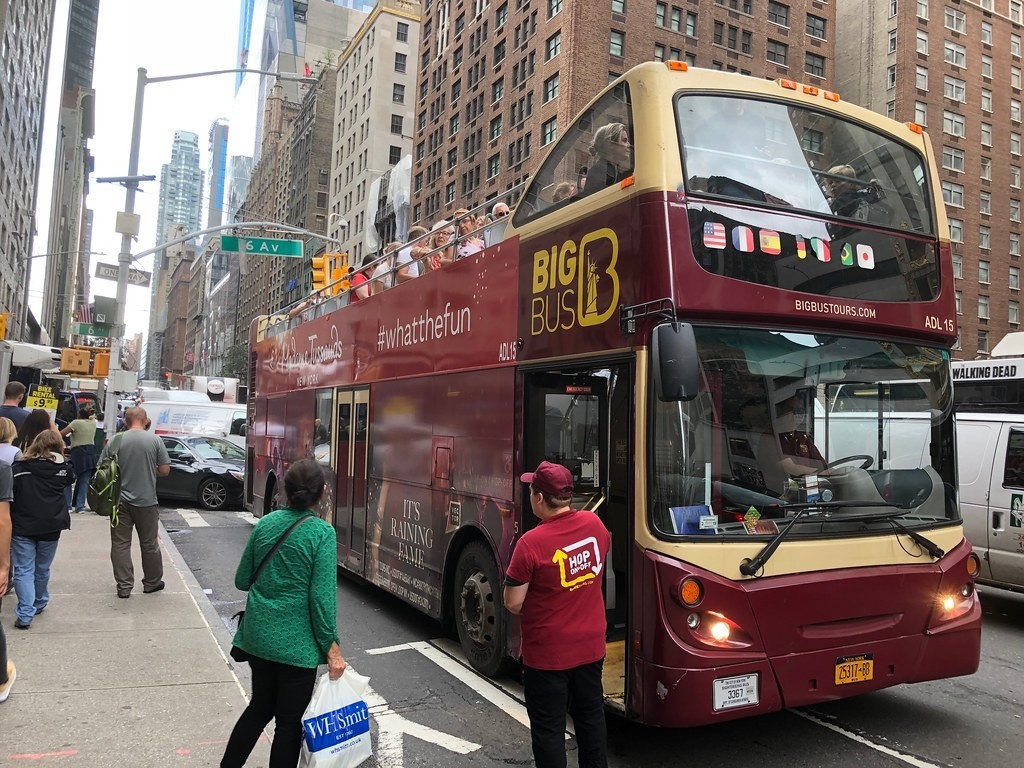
229, 611, 248, 662
299, 667, 372, 768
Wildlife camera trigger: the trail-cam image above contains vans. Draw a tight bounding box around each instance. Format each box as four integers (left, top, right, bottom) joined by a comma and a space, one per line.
134, 400, 248, 451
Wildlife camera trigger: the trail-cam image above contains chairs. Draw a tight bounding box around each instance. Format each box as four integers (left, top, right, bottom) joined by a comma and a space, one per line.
265, 194, 550, 338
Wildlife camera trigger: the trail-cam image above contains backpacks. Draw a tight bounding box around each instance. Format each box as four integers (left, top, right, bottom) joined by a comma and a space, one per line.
87, 432, 123, 528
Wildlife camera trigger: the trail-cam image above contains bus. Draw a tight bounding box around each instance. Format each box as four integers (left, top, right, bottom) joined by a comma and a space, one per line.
814, 331, 1024, 595
242, 64, 983, 729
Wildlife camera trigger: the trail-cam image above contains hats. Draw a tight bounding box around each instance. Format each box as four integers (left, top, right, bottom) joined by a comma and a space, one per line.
520, 461, 574, 497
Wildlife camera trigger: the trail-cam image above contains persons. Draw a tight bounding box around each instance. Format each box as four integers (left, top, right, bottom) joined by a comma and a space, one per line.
818, 165, 868, 222
584, 123, 630, 191
395, 225, 428, 286
504, 461, 609, 768
552, 182, 576, 203
60, 409, 97, 513
440, 208, 485, 265
97, 407, 170, 597
759, 398, 832, 493
350, 254, 380, 302
290, 290, 322, 323
219, 460, 347, 768
117, 400, 141, 431
371, 242, 403, 296
492, 203, 510, 218
314, 419, 327, 443
96, 413, 104, 428
411, 220, 455, 271
477, 216, 492, 239
0, 381, 75, 701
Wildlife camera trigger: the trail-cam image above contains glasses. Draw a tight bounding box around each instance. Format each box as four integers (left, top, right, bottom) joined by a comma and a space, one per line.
437, 231, 454, 238
461, 219, 472, 224
494, 212, 509, 217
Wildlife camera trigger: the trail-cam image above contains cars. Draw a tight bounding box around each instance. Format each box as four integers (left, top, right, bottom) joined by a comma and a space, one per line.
154, 432, 247, 511
52, 375, 214, 444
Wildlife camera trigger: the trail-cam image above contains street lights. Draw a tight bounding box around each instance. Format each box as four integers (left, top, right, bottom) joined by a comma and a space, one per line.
102, 66, 319, 430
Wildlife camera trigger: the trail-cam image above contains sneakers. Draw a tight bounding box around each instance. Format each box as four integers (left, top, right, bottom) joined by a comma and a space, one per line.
0, 660, 16, 702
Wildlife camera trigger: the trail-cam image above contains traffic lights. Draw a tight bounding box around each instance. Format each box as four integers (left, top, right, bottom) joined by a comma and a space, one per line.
163, 368, 172, 382
309, 257, 329, 297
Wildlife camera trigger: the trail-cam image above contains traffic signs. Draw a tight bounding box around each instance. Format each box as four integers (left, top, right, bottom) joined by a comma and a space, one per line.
95, 262, 152, 287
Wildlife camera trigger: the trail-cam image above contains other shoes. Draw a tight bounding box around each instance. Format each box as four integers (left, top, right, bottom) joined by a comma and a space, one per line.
77, 508, 86, 513
68, 506, 73, 513
118, 592, 129, 598
144, 581, 164, 593
15, 620, 30, 630
